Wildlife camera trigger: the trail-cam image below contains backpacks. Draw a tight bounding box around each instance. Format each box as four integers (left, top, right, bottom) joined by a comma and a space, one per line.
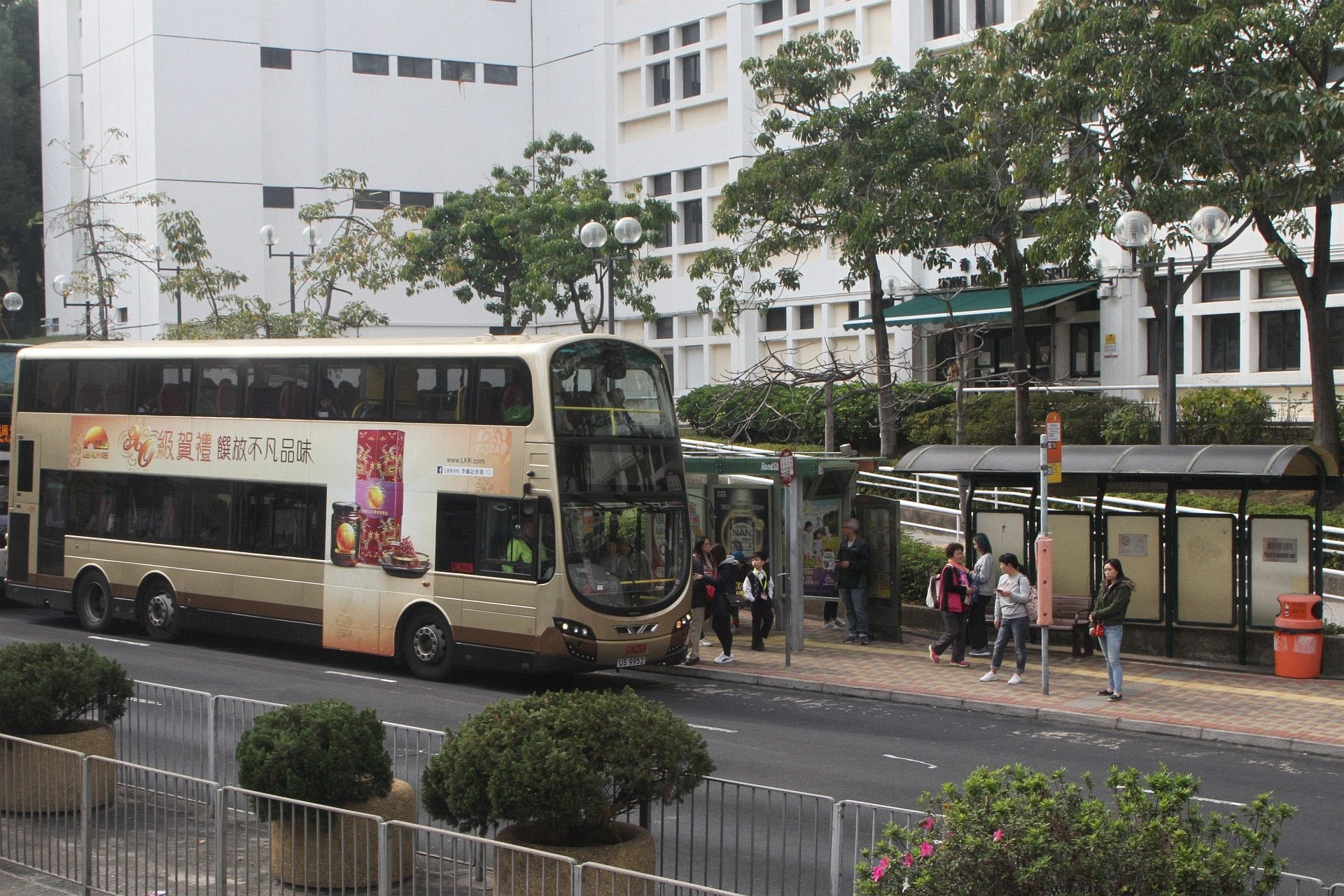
926, 574, 940, 609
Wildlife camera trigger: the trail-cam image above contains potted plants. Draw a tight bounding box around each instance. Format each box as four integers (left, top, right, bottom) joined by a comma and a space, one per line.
234, 697, 418, 889
421, 685, 718, 895
966, 592, 1094, 660
1, 639, 135, 814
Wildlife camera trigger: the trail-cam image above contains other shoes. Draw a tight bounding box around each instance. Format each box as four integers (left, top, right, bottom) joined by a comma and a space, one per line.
732, 628, 742, 634
1108, 694, 1122, 702
1096, 690, 1114, 696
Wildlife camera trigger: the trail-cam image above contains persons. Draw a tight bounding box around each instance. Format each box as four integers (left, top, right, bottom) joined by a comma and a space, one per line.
979, 552, 1030, 685
683, 535, 775, 665
741, 551, 775, 652
1088, 558, 1138, 703
928, 542, 975, 668
834, 517, 873, 645
964, 532, 996, 656
822, 601, 845, 631
801, 520, 824, 568
40, 364, 653, 602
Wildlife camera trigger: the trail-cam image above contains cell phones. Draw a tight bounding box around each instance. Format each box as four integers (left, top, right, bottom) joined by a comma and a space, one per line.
836, 560, 841, 563
994, 589, 1002, 592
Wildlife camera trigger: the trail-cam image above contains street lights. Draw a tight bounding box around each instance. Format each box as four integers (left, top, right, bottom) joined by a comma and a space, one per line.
579, 217, 643, 337
53, 273, 119, 340
260, 224, 323, 339
1114, 205, 1230, 444
149, 242, 198, 327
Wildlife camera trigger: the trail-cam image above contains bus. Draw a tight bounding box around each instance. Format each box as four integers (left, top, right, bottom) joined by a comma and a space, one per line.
8, 332, 696, 689
0, 342, 37, 600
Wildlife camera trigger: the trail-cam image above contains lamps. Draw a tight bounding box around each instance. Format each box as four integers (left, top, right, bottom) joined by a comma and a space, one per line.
1098, 286, 1113, 300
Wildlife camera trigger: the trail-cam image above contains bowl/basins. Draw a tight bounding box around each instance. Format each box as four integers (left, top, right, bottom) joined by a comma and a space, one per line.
378, 559, 433, 579
383, 550, 430, 569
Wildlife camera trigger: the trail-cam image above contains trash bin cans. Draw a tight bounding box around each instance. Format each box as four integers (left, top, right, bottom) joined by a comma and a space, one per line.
1273, 594, 1325, 680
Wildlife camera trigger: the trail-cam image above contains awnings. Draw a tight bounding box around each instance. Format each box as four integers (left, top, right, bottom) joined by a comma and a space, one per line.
839, 280, 1103, 331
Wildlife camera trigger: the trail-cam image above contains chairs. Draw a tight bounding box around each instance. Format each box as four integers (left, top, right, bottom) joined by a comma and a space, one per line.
53, 378, 611, 431
42, 483, 320, 560
491, 523, 513, 559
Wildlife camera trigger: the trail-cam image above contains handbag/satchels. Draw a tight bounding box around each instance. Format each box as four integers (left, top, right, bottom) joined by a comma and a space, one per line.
727, 594, 745, 608
1025, 586, 1038, 620
1095, 624, 1104, 637
970, 583, 978, 602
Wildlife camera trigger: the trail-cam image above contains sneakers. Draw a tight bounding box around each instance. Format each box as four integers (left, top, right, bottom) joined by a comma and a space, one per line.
823, 622, 840, 630
950, 661, 969, 667
969, 649, 990, 656
834, 618, 845, 628
1008, 673, 1023, 684
928, 644, 940, 664
699, 638, 712, 647
685, 658, 699, 666
714, 653, 732, 663
980, 671, 997, 681
859, 637, 869, 644
843, 637, 860, 643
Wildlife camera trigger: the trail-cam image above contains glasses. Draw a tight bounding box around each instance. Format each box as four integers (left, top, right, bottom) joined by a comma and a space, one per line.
842, 526, 852, 529
